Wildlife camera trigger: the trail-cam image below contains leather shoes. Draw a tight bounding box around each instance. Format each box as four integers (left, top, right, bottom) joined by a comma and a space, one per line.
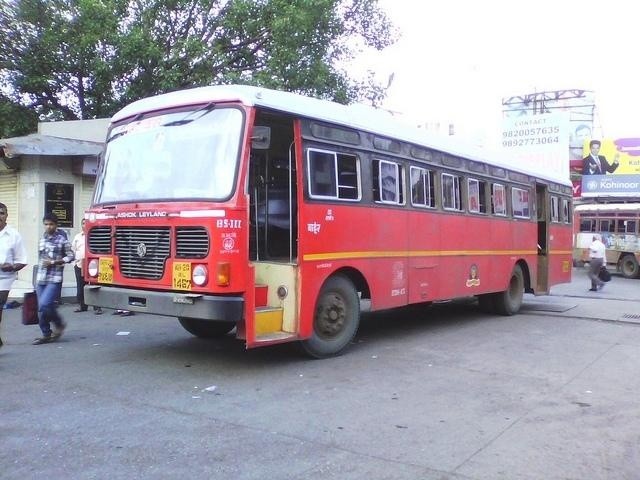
112, 311, 135, 316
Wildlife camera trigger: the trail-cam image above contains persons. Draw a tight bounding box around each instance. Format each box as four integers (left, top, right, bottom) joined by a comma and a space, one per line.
32, 212, 75, 344
71, 217, 103, 313
113, 309, 135, 316
571, 125, 592, 158
583, 140, 620, 174
587, 233, 607, 291
0, 203, 26, 347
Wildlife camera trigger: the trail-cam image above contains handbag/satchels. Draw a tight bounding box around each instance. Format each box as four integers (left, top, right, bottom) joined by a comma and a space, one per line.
23, 290, 38, 325
599, 266, 611, 283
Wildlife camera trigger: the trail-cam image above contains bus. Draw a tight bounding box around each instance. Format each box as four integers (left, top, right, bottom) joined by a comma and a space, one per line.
572, 203, 640, 279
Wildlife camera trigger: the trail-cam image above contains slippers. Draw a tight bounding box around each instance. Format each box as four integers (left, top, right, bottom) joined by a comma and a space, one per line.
32, 335, 52, 344
74, 307, 82, 312
51, 321, 66, 341
95, 309, 102, 314
